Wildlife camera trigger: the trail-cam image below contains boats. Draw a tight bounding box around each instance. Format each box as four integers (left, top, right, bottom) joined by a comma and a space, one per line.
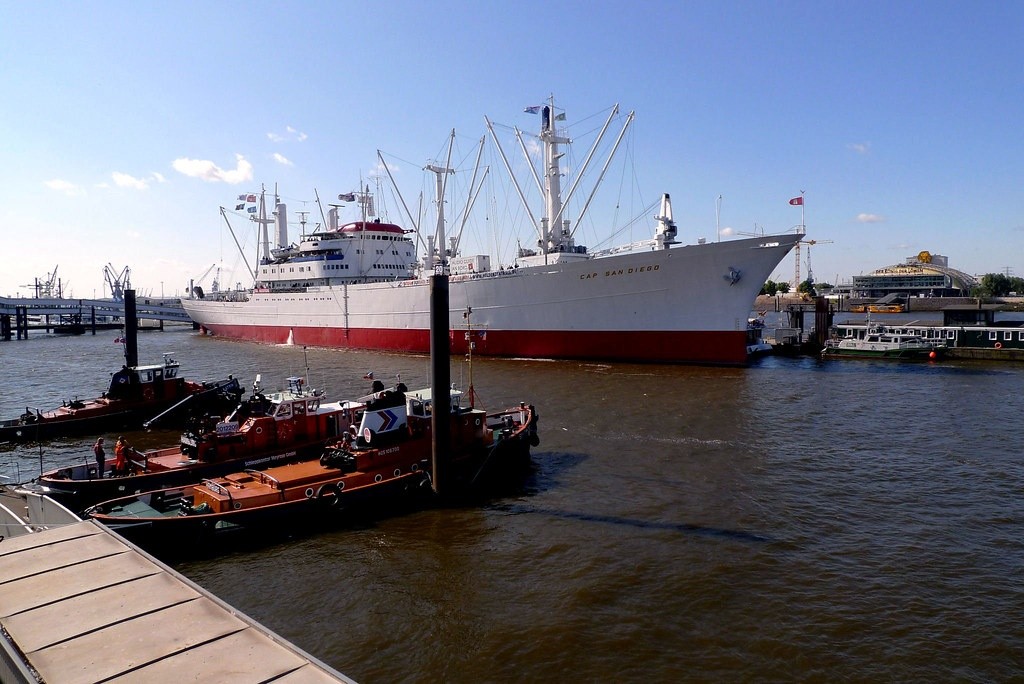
857, 306, 903, 313
0, 351, 246, 441
37, 372, 406, 496
819, 332, 949, 361
838, 299, 1024, 347
82, 384, 542, 555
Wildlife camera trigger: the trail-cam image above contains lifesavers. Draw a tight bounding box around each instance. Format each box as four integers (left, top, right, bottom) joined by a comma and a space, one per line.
316, 483, 343, 509
143, 388, 155, 402
994, 342, 1002, 350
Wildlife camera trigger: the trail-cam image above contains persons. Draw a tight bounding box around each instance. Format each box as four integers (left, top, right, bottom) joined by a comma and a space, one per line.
93, 437, 106, 479
114, 434, 130, 474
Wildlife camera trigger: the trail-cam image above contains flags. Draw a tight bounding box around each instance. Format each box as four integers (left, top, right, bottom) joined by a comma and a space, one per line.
524, 106, 541, 115
338, 193, 356, 203
247, 195, 256, 202
247, 206, 257, 214
113, 337, 126, 344
789, 196, 803, 206
554, 112, 567, 121
235, 203, 245, 211
364, 371, 374, 381
236, 194, 247, 201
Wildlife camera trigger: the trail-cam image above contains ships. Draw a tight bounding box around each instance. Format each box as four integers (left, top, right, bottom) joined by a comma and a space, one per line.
178, 93, 806, 368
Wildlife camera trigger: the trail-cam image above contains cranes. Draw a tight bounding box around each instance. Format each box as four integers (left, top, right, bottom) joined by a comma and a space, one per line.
734, 224, 835, 297
